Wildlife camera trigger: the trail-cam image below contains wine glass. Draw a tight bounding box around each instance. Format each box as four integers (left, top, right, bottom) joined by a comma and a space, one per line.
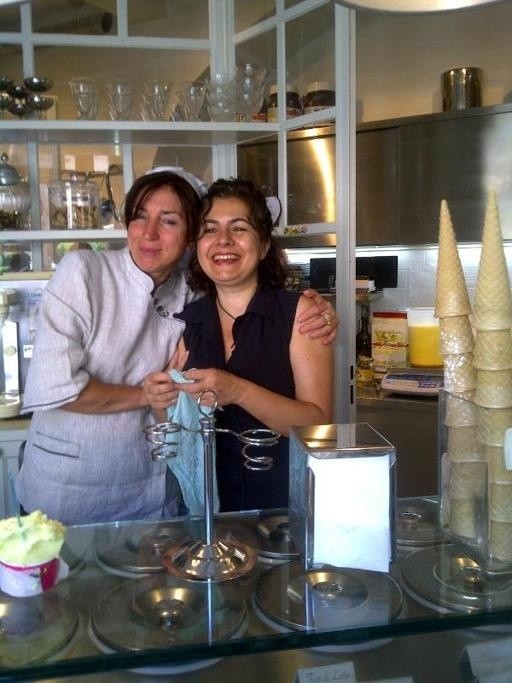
67, 77, 205, 121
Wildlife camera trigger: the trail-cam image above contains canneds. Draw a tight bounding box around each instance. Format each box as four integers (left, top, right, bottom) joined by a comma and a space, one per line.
237, 81, 335, 123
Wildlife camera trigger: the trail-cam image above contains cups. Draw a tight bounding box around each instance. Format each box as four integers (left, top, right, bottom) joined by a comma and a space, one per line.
442, 65, 480, 112
407, 306, 443, 367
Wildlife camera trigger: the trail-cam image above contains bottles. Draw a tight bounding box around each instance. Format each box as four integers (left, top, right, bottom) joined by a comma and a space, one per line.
47, 172, 101, 230
357, 316, 371, 364
0, 152, 32, 230
268, 81, 336, 122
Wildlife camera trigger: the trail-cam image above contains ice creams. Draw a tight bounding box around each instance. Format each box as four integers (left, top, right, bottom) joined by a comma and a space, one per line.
0, 510, 67, 566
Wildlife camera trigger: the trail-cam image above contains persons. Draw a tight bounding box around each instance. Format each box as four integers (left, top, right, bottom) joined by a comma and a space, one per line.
15, 164, 340, 524
137, 174, 338, 544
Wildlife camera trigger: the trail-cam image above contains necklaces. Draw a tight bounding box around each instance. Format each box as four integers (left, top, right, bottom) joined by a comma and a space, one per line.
216, 292, 239, 321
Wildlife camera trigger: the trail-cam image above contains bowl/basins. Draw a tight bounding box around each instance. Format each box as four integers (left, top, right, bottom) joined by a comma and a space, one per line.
204, 64, 266, 122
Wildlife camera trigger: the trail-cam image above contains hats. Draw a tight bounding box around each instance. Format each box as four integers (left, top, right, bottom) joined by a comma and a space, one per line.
145, 162, 207, 201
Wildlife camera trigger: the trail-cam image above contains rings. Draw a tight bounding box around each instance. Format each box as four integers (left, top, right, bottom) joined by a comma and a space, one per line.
323, 312, 332, 324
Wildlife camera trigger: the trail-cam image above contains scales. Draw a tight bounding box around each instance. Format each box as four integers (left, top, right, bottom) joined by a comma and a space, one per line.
381, 362, 444, 396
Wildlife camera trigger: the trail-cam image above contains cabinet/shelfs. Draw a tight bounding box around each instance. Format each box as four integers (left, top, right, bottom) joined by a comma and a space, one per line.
0, 1, 354, 421
